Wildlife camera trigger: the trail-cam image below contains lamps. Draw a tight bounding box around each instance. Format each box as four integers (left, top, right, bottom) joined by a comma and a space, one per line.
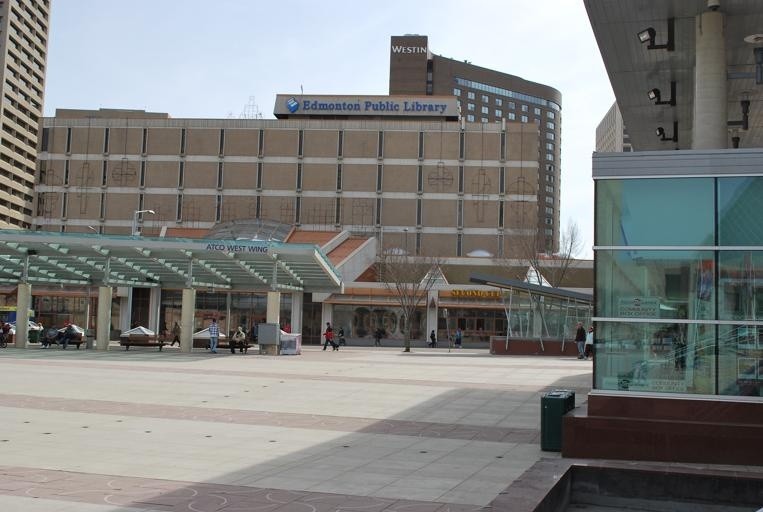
648, 88, 671, 107
637, 28, 667, 50
655, 127, 674, 142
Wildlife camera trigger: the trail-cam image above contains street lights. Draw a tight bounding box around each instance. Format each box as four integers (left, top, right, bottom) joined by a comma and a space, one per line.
134, 209, 156, 234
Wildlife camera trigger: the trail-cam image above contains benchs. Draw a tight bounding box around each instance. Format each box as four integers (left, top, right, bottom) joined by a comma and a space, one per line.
208, 337, 251, 354
120, 335, 167, 351
41, 334, 82, 349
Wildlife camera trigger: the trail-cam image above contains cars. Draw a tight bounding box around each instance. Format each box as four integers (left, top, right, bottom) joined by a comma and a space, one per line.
28, 321, 44, 331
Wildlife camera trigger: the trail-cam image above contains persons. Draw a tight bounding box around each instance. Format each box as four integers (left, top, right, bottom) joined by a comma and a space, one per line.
36, 321, 59, 350
55, 324, 76, 349
453, 327, 462, 349
372, 328, 382, 347
667, 305, 689, 372
208, 318, 221, 354
228, 326, 246, 355
169, 321, 180, 347
572, 322, 587, 360
321, 321, 337, 352
336, 326, 345, 346
582, 324, 594, 361
427, 329, 436, 348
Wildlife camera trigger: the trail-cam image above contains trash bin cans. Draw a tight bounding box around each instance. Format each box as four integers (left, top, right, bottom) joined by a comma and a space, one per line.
29, 330, 40, 343
541, 389, 575, 452
87, 336, 93, 349
